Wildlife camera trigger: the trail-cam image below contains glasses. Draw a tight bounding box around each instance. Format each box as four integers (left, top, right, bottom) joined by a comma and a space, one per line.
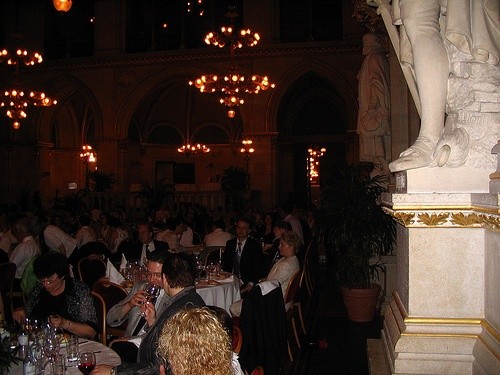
38, 276, 59, 286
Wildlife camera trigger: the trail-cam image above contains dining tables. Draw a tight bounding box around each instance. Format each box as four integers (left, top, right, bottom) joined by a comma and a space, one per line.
0, 333, 123, 375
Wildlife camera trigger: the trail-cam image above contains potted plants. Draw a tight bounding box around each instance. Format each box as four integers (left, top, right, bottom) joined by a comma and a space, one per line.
313, 161, 406, 323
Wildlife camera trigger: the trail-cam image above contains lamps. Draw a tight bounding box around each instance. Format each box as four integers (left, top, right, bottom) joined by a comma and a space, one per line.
204, 12, 261, 52
240, 137, 254, 155
307, 146, 326, 180
80, 144, 97, 165
188, 64, 275, 118
0, 84, 58, 130
0, 44, 44, 69
177, 137, 210, 159
53, 0, 72, 14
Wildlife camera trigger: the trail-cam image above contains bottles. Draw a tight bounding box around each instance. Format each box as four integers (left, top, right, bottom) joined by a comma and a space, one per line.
260, 235, 264, 248
17, 319, 58, 375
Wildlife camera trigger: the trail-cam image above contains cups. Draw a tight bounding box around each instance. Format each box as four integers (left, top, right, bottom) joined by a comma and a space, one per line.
196, 255, 200, 267
53, 354, 66, 375
77, 351, 97, 375
66, 334, 78, 361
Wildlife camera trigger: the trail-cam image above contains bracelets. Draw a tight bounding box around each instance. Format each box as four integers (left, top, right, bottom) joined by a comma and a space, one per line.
66, 321, 71, 331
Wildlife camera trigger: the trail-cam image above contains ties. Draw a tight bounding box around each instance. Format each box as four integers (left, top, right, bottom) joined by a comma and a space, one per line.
237, 242, 242, 253
145, 245, 151, 259
132, 296, 157, 336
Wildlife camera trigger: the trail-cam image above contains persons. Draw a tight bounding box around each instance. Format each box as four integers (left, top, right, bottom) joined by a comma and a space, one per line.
265, 221, 292, 263
223, 218, 262, 294
125, 223, 170, 262
90, 253, 205, 375
72, 226, 107, 261
293, 207, 312, 245
12, 251, 98, 341
279, 205, 304, 247
240, 231, 299, 312
155, 307, 234, 375
36, 217, 78, 264
107, 250, 171, 362
357, 33, 388, 179
248, 212, 274, 243
388, 0, 447, 171
0, 218, 40, 293
73, 209, 111, 250
205, 306, 245, 375
157, 216, 232, 252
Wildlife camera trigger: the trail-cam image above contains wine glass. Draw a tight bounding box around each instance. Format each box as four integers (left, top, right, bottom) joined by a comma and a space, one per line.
126, 264, 136, 281
216, 260, 222, 277
136, 282, 159, 317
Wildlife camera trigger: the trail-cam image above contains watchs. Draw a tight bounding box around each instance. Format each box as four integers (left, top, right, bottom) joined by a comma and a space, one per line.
110, 366, 115, 375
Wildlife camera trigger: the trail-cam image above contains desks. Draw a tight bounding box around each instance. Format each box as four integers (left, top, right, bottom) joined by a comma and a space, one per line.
116, 267, 242, 321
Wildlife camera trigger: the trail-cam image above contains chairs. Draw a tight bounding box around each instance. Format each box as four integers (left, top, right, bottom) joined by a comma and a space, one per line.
0, 221, 320, 375
